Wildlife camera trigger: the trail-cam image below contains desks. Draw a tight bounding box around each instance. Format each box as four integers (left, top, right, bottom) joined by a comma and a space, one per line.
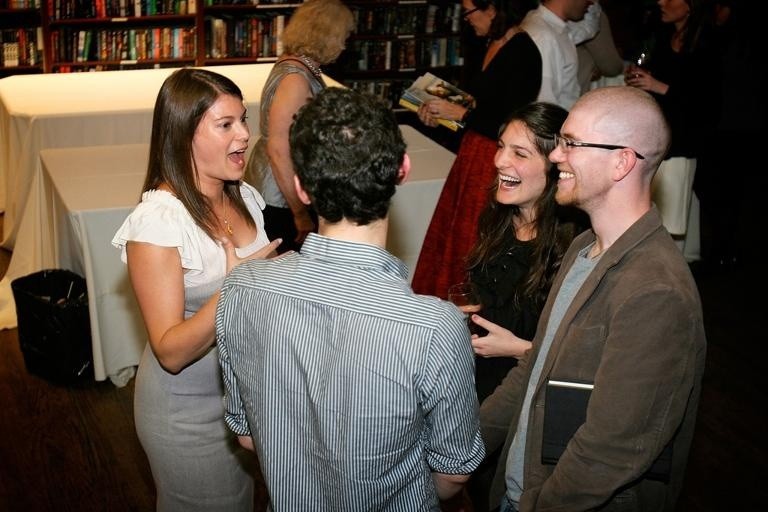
0, 63, 349, 253
1, 124, 458, 386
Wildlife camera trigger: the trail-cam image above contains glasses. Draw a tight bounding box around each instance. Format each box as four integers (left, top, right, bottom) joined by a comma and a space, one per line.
553, 133, 645, 159
461, 8, 480, 18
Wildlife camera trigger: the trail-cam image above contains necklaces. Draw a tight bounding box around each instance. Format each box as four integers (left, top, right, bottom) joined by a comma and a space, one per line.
215, 193, 235, 236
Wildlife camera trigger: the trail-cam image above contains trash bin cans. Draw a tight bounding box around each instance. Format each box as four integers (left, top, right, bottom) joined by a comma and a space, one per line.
11, 269, 94, 384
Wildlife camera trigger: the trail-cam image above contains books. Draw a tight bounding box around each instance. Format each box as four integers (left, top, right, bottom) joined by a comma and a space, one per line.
0, 25, 197, 67
202, 14, 286, 59
339, 0, 465, 70
399, 72, 475, 132
0, 0, 198, 20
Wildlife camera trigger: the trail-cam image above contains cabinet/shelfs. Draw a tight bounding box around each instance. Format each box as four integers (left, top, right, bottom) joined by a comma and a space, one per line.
0, 1, 484, 117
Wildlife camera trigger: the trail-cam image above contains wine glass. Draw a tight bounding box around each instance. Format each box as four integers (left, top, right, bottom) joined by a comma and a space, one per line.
624, 53, 649, 86
448, 284, 483, 327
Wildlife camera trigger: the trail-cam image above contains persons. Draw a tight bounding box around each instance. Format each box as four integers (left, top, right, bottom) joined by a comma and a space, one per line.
243, 1, 358, 255
520, 0, 602, 113
475, 86, 708, 512
112, 67, 283, 512
623, 1, 712, 265
577, 11, 634, 96
455, 101, 585, 405
214, 86, 486, 512
411, 0, 542, 304
